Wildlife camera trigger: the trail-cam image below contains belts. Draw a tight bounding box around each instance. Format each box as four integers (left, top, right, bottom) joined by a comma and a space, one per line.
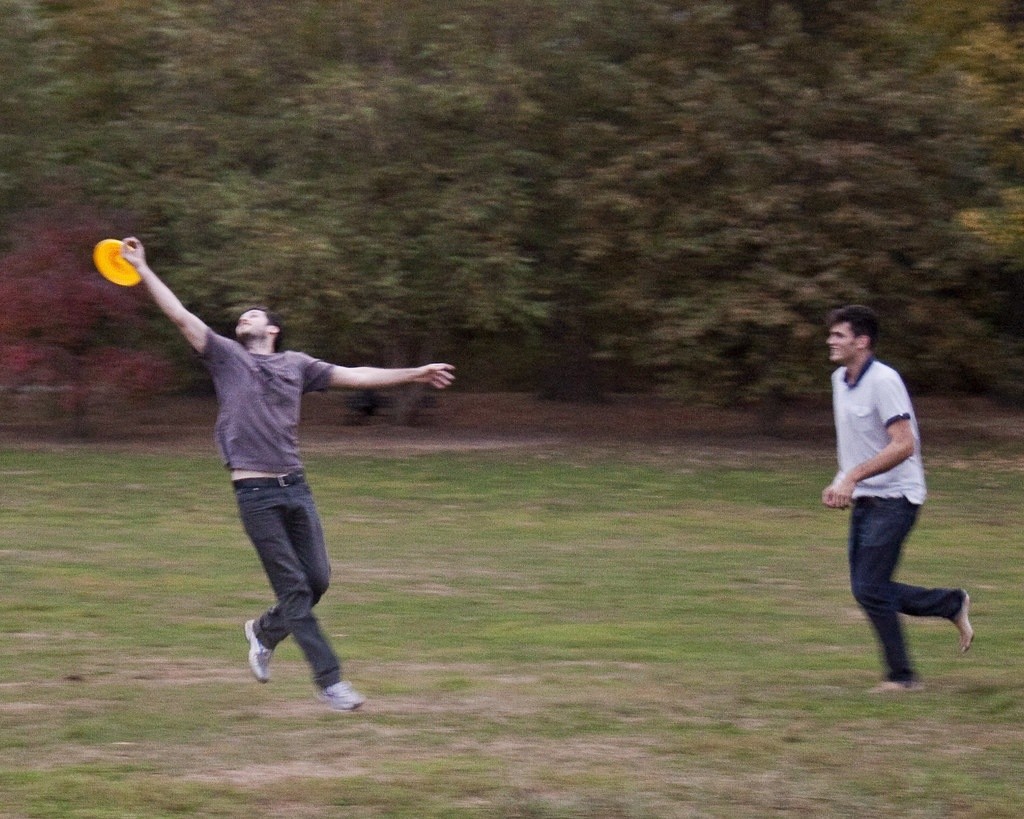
232, 474, 305, 490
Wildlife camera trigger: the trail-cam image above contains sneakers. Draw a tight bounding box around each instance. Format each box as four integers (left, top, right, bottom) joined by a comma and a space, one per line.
312, 675, 365, 712
245, 619, 270, 682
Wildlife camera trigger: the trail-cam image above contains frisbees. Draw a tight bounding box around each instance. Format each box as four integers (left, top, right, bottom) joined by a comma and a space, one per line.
93, 238, 143, 288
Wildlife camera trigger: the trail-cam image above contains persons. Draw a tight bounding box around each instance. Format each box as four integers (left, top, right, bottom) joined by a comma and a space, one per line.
820, 305, 973, 693
121, 237, 456, 711
